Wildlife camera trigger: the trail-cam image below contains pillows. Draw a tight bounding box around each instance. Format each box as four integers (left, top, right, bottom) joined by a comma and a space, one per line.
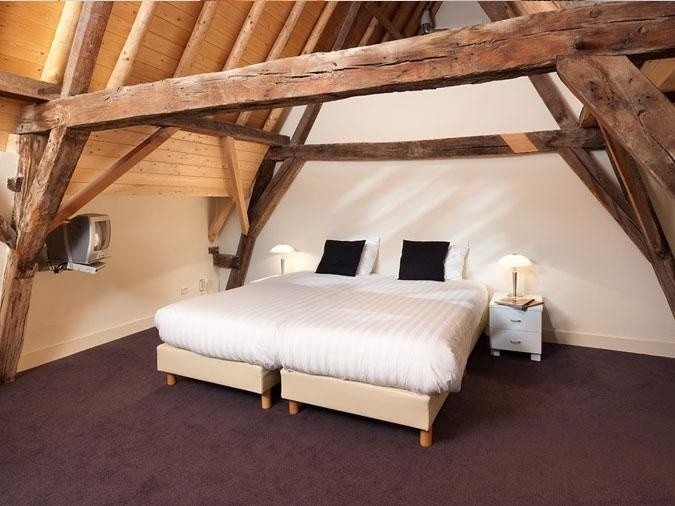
343, 238, 380, 276
394, 240, 470, 280
315, 239, 366, 277
398, 240, 450, 282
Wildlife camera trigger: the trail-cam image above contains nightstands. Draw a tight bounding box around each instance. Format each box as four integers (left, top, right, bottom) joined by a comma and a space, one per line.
488, 293, 544, 362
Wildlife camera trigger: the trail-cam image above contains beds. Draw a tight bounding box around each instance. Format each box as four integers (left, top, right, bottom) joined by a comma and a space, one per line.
154, 275, 489, 448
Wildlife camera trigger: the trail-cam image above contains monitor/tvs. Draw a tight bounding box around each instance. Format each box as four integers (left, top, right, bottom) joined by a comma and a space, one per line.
45, 213, 112, 264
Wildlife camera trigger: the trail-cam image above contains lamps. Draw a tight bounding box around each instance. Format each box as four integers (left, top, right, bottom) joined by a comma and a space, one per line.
269, 244, 297, 275
497, 253, 532, 299
